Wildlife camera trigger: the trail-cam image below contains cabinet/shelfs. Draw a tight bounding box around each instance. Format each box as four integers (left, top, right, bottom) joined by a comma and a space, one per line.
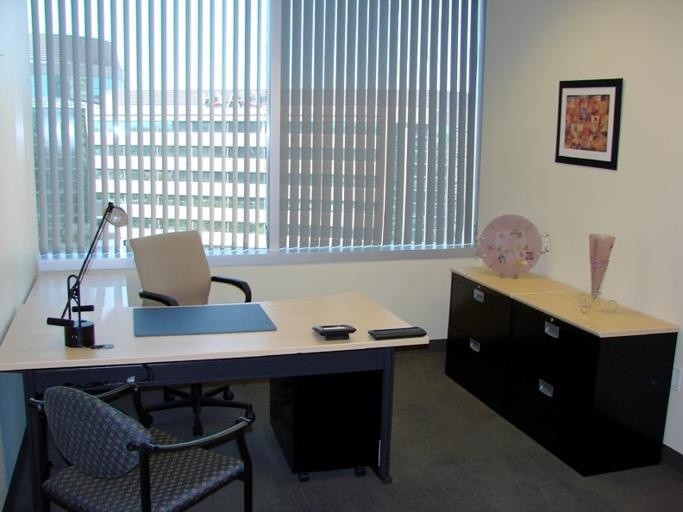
443, 267, 678, 477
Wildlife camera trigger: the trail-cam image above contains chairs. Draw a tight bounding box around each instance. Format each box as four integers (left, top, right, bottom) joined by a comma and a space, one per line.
127, 231, 252, 437
28, 380, 255, 511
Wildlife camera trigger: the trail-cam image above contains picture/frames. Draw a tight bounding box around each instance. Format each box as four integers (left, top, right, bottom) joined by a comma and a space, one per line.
555, 78, 624, 170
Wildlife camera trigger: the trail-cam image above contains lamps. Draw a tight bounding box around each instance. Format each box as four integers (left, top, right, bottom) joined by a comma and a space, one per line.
47, 202, 128, 349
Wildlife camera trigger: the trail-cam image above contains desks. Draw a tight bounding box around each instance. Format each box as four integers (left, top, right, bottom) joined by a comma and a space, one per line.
5, 292, 430, 511
